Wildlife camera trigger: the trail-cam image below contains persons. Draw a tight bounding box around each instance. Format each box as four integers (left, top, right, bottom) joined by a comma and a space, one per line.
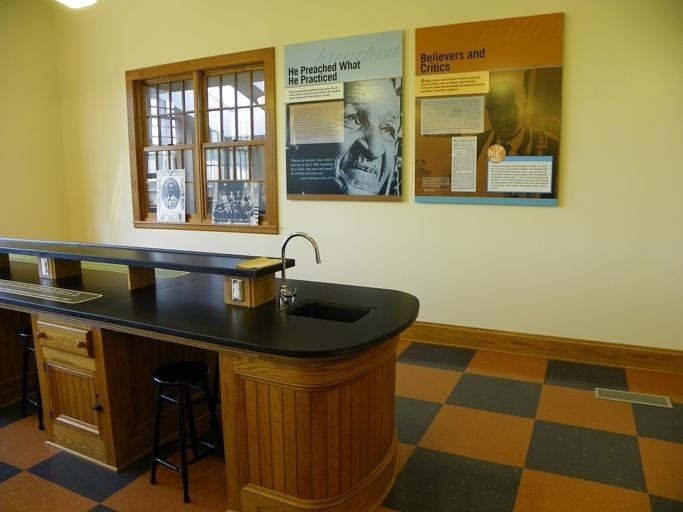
477, 79, 558, 199
331, 78, 401, 197
213, 190, 254, 224
162, 182, 178, 209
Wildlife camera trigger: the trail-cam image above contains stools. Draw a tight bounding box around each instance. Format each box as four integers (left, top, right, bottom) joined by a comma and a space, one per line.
15, 327, 45, 430
150, 361, 224, 503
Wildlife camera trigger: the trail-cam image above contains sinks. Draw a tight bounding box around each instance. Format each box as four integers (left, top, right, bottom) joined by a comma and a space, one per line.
284, 299, 373, 324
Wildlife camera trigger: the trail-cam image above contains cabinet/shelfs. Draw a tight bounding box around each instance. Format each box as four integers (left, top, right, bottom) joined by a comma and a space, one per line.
31, 313, 119, 474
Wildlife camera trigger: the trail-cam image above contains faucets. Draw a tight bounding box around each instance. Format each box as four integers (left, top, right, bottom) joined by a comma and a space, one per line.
280, 233, 322, 308
